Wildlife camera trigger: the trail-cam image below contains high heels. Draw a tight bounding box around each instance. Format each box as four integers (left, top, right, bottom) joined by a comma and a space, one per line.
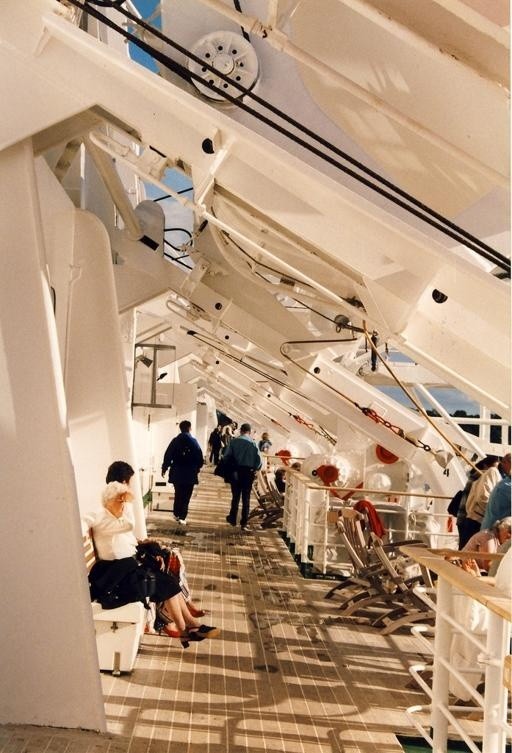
159, 610, 221, 648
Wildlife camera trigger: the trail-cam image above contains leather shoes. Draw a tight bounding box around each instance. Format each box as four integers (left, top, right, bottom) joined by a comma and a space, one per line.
226, 516, 237, 526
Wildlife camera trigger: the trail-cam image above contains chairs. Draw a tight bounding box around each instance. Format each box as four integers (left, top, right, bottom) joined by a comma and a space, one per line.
246, 465, 285, 529
325, 504, 474, 637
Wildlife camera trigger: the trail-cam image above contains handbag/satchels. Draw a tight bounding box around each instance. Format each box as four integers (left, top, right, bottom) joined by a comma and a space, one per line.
100, 569, 147, 609
447, 490, 463, 517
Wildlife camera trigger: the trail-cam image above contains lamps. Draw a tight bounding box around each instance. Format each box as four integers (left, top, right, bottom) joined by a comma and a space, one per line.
158, 369, 168, 383
135, 353, 152, 367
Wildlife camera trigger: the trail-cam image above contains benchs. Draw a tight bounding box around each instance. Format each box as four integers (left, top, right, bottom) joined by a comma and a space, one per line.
83, 532, 149, 673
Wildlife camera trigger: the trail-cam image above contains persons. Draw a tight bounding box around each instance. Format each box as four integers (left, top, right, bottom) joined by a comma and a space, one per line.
207, 420, 302, 500
105, 461, 205, 639
455, 456, 503, 533
160, 420, 204, 525
480, 474, 512, 528
224, 422, 262, 526
457, 452, 511, 551
84, 480, 222, 649
462, 515, 512, 578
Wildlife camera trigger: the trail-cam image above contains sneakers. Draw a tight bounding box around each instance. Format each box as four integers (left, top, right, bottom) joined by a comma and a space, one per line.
173, 514, 187, 525
